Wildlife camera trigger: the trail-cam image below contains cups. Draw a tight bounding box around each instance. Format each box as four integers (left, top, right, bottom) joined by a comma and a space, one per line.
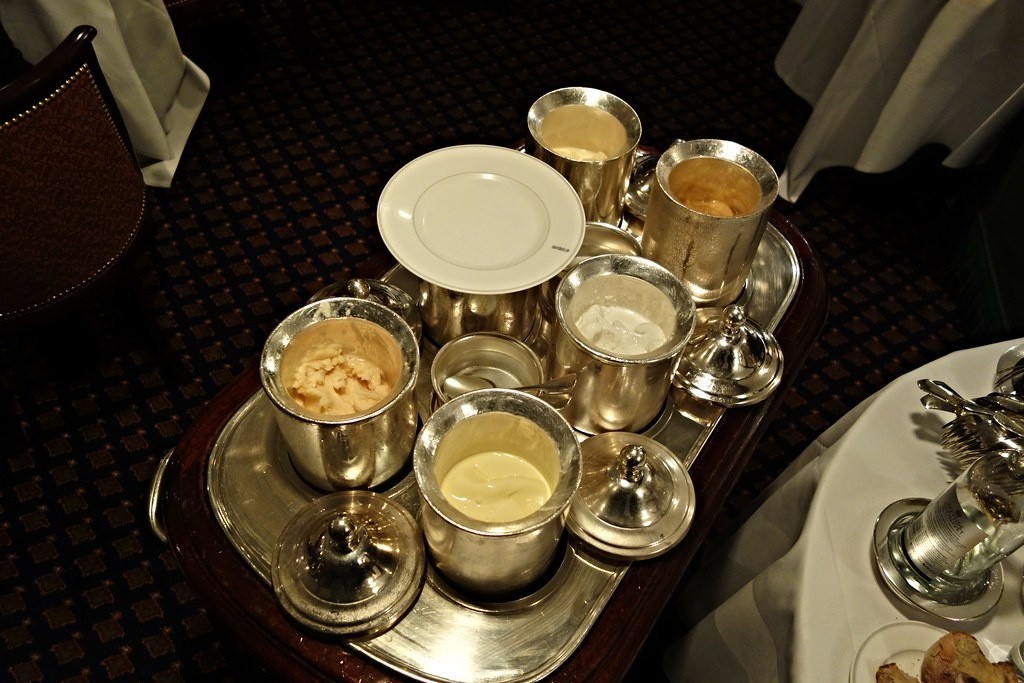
260, 299, 419, 495
413, 386, 583, 602
517, 87, 641, 226
546, 252, 697, 437
642, 133, 779, 311
433, 332, 548, 410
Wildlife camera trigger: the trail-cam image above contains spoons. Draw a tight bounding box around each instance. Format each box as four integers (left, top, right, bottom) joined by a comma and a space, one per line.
444, 373, 576, 400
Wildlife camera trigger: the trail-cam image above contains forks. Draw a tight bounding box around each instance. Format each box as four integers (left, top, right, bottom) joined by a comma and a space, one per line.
916, 359, 1024, 470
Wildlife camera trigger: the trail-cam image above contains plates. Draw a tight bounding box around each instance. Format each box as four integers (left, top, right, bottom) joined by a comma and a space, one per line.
376, 144, 586, 294
849, 621, 994, 683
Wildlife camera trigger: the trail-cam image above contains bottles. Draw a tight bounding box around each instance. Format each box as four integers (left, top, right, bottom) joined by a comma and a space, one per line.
905, 443, 1024, 598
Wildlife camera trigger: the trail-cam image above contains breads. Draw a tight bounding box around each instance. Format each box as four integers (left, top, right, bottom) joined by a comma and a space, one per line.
876, 632, 1018, 683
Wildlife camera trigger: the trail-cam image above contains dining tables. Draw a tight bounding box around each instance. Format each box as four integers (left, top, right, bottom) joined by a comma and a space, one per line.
789, 338, 1024, 683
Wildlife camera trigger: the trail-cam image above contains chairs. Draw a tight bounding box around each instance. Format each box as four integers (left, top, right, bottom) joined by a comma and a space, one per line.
1, 24, 148, 362
